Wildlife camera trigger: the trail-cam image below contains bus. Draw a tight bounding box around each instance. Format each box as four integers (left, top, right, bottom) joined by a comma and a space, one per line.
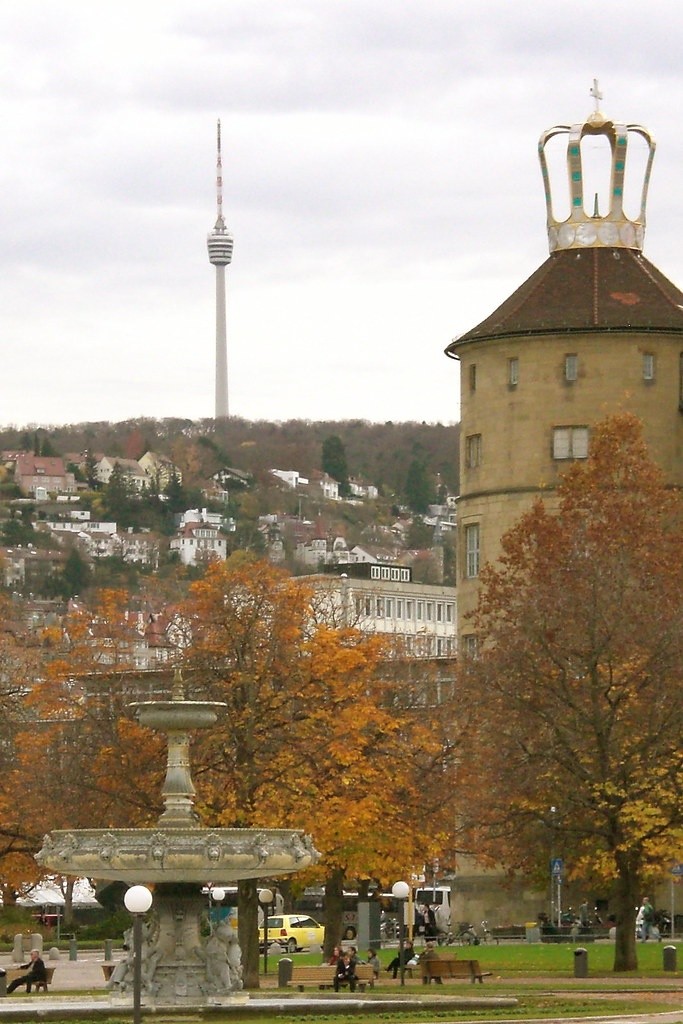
286, 886, 410, 940
201, 886, 286, 928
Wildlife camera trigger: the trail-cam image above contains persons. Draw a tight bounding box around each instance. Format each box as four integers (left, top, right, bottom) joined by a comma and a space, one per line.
329, 946, 343, 966
413, 908, 421, 937
359, 948, 380, 991
579, 898, 588, 922
384, 939, 414, 979
640, 896, 661, 943
421, 904, 437, 943
6, 949, 46, 994
418, 941, 443, 985
333, 952, 357, 992
348, 947, 360, 963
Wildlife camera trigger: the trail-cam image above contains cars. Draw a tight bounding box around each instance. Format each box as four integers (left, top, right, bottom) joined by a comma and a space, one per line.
257, 914, 325, 952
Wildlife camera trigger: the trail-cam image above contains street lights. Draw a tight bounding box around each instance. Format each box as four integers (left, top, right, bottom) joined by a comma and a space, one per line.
211, 888, 227, 919
391, 880, 411, 987
258, 888, 275, 975
123, 885, 153, 1024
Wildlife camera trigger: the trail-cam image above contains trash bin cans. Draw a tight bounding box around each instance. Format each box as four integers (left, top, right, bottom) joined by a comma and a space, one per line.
574, 948, 588, 977
663, 944, 676, 973
278, 958, 293, 988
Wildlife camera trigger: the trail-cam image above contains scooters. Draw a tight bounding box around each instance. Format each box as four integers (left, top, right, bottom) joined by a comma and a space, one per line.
534, 902, 675, 942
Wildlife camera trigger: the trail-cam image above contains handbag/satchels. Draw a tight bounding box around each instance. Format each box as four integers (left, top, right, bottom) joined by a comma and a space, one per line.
407, 955, 419, 965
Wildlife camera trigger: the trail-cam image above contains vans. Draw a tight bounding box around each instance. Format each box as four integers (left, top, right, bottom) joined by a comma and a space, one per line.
414, 885, 450, 932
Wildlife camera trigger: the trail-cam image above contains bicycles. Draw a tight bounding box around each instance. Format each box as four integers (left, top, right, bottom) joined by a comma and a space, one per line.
460, 919, 500, 947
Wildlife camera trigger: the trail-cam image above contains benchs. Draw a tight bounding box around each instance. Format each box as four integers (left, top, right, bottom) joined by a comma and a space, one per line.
287, 965, 374, 993
419, 959, 492, 985
5, 968, 56, 992
